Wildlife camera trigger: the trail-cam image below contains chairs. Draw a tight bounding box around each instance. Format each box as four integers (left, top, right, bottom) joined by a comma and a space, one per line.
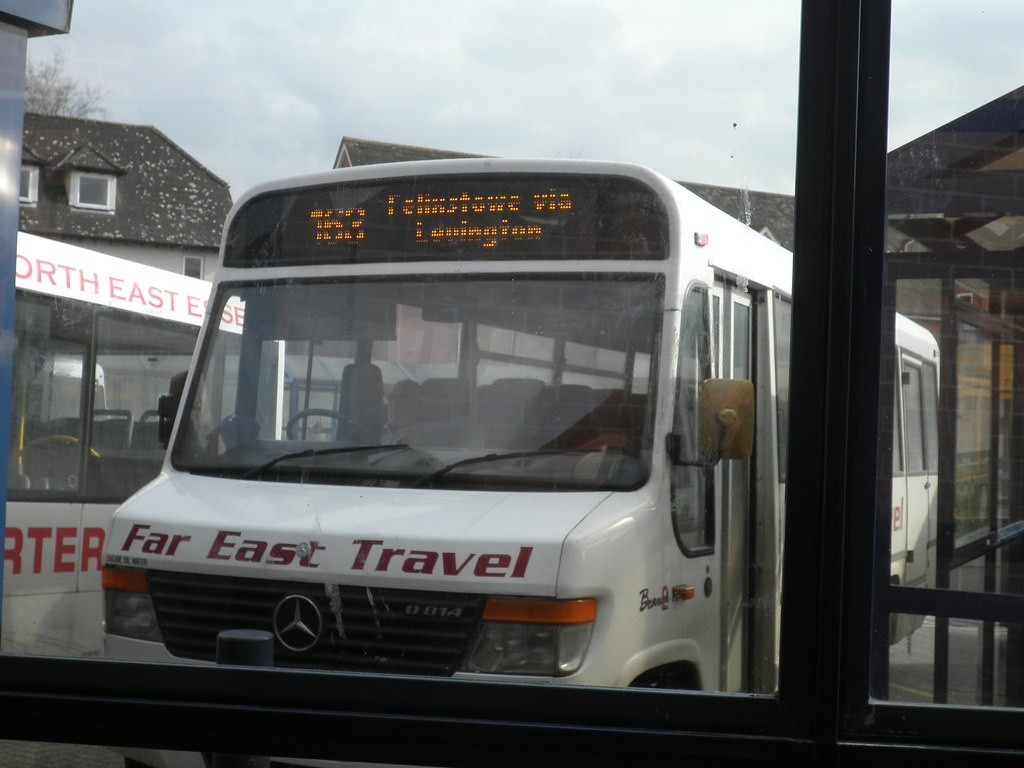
412, 376, 649, 455
41, 408, 171, 457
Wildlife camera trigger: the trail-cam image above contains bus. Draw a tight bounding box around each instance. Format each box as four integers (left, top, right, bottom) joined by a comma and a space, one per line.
0, 230, 284, 669
100, 158, 943, 693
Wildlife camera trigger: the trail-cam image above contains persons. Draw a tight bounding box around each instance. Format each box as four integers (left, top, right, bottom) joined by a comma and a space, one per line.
375, 379, 450, 449
